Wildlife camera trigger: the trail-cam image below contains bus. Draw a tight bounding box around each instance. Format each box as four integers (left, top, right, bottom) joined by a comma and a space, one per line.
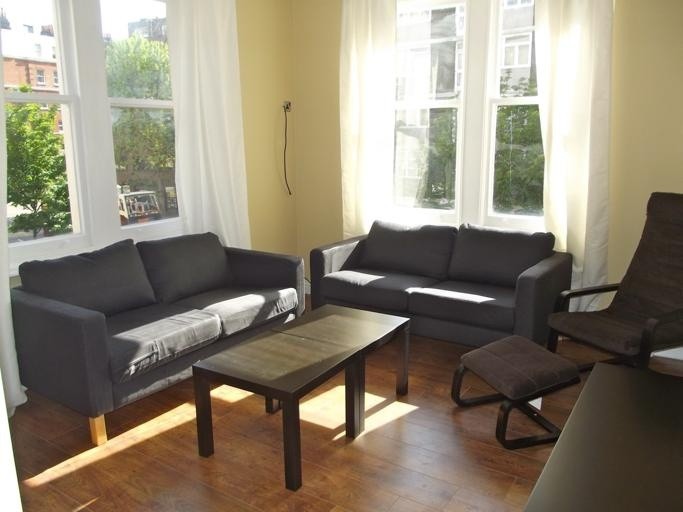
116, 189, 161, 225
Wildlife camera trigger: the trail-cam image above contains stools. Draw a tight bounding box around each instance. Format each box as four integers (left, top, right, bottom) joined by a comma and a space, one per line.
451, 333, 582, 451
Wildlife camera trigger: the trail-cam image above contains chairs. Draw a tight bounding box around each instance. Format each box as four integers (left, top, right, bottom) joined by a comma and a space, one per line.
544, 192, 683, 370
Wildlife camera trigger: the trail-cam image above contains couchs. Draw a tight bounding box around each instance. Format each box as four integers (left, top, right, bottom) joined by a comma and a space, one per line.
9, 231, 307, 444
310, 218, 573, 348
